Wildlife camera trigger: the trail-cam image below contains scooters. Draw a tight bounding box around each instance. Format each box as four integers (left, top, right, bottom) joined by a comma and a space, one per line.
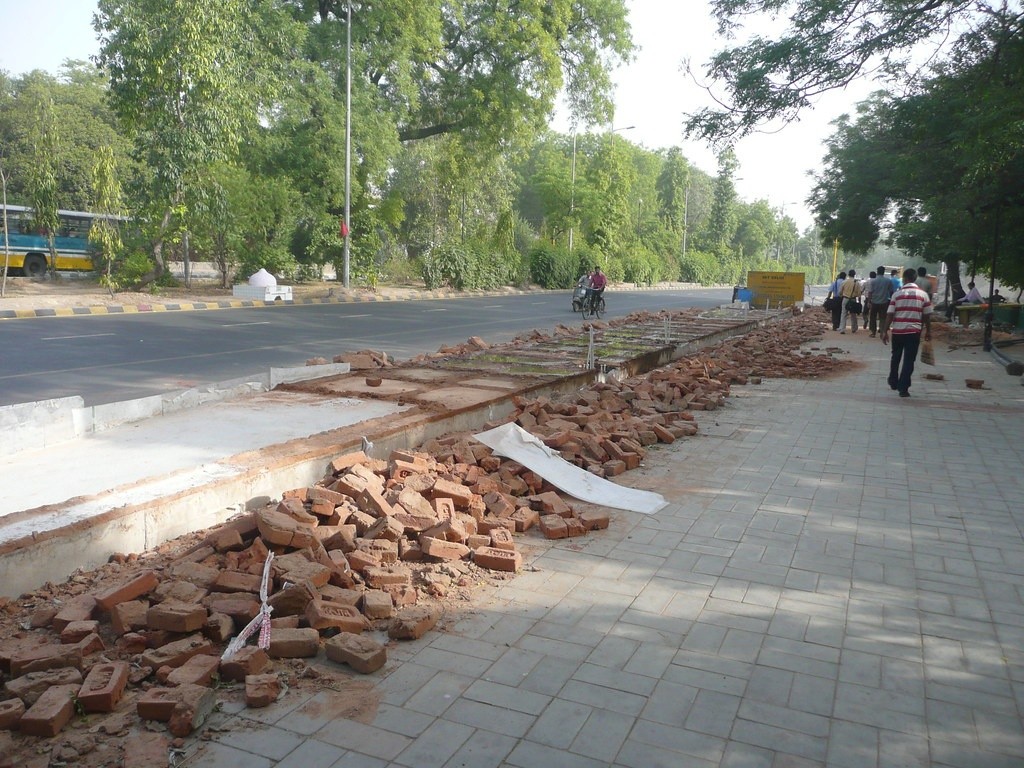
571, 285, 592, 312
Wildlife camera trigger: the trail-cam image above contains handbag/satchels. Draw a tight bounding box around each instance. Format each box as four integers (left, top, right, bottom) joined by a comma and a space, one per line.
824, 299, 834, 311
845, 300, 862, 314
921, 340, 934, 365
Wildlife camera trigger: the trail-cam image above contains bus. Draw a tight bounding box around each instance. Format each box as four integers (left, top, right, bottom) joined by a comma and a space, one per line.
0, 202, 155, 277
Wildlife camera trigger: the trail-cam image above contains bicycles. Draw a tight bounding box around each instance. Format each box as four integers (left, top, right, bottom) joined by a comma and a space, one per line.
582, 287, 607, 319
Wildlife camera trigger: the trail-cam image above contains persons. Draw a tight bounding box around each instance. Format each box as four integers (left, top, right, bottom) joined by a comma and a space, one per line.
576, 265, 607, 315
826, 266, 1007, 338
882, 269, 933, 397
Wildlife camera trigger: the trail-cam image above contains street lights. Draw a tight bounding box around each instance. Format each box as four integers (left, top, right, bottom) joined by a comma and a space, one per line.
605, 124, 636, 263
773, 202, 798, 263
637, 199, 643, 246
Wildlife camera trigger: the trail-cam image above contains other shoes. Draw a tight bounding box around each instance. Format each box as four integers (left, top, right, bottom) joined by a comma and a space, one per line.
943, 318, 951, 323
864, 321, 868, 329
869, 334, 876, 337
899, 392, 911, 397
841, 332, 845, 334
888, 377, 897, 390
